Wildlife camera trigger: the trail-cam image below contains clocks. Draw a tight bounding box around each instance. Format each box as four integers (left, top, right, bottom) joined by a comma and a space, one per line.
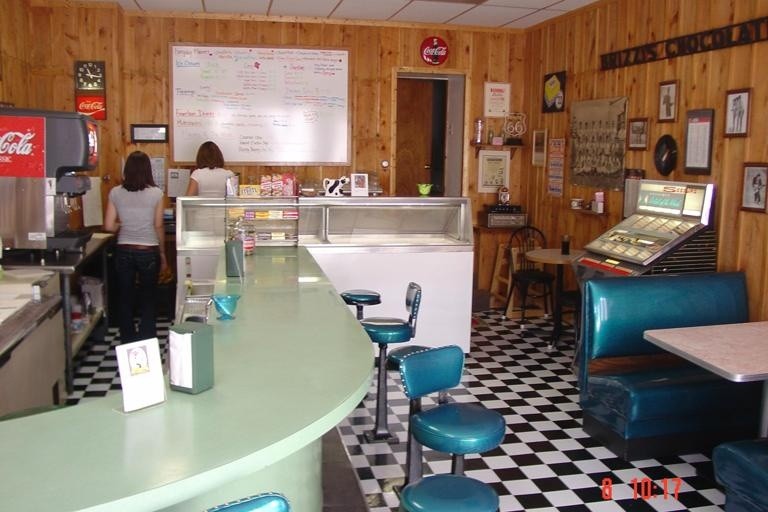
75, 61, 105, 91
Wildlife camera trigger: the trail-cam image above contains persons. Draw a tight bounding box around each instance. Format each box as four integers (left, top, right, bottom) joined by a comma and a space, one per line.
727, 96, 738, 132
185, 142, 237, 197
572, 115, 623, 180
103, 150, 169, 350
751, 174, 765, 205
661, 85, 675, 117
735, 94, 745, 133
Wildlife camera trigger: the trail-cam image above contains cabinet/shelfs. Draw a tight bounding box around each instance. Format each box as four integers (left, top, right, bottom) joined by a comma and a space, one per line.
224, 183, 299, 248
472, 143, 528, 233
176, 196, 475, 358
0, 233, 117, 394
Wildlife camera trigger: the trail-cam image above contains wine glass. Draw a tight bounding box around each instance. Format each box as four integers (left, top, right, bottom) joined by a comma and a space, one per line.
416, 183, 433, 197
208, 293, 240, 321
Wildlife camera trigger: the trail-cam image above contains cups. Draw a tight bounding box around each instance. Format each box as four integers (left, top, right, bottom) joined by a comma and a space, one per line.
560, 234, 572, 254
322, 176, 350, 198
225, 239, 245, 278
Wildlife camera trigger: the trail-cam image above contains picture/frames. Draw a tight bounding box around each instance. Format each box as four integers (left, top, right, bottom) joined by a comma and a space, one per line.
626, 79, 768, 213
130, 124, 169, 143
477, 150, 510, 193
483, 81, 511, 119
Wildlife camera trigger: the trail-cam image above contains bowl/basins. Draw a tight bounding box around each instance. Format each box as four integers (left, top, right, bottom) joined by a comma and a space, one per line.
301, 190, 318, 197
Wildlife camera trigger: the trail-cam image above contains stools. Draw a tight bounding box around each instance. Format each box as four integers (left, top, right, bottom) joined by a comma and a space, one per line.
339, 289, 381, 319
387, 347, 447, 501
400, 474, 499, 511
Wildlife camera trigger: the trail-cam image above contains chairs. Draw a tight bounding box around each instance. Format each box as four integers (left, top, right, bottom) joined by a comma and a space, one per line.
204, 492, 293, 512
402, 346, 508, 476
361, 283, 422, 445
490, 226, 586, 350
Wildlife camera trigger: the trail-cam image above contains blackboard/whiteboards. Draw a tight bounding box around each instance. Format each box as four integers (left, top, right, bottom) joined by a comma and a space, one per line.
167, 41, 352, 168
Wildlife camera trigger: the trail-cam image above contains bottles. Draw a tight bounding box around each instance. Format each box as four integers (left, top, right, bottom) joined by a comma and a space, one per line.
471, 118, 485, 144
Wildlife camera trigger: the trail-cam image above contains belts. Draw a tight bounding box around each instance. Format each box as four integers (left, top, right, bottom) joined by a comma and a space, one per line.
120, 244, 158, 250
